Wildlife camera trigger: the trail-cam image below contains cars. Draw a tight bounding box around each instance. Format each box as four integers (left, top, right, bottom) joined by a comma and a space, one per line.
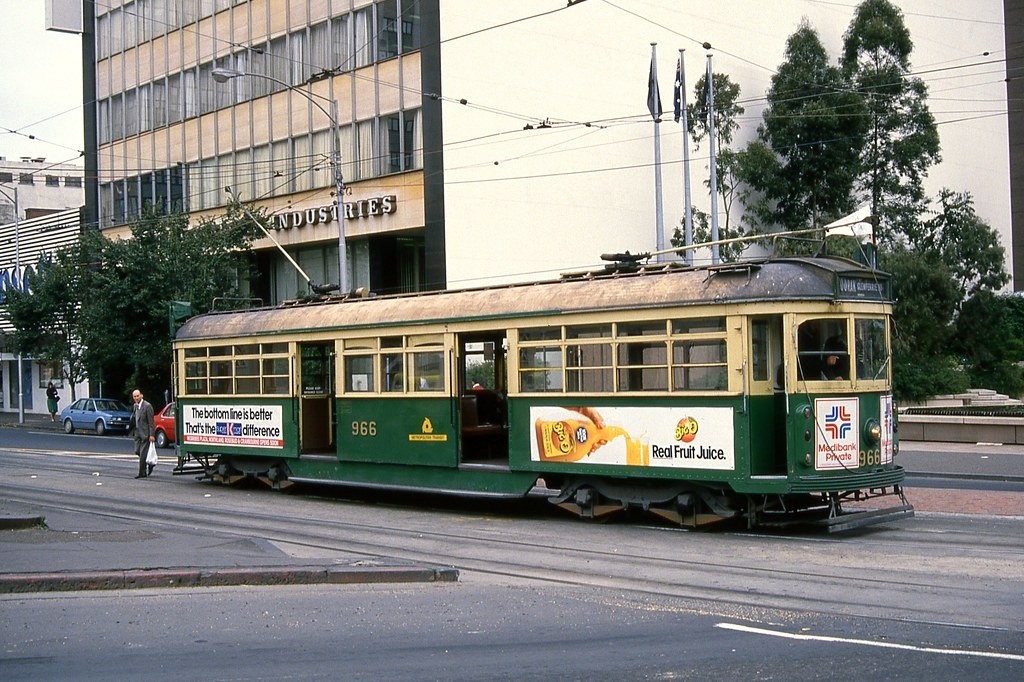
154, 402, 176, 449
59, 398, 135, 435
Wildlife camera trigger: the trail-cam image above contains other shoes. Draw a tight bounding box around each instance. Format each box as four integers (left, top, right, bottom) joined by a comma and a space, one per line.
135, 474, 147, 479
52, 419, 55, 423
148, 464, 156, 475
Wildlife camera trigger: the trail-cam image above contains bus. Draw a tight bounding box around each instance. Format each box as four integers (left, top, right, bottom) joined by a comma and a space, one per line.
171, 214, 914, 534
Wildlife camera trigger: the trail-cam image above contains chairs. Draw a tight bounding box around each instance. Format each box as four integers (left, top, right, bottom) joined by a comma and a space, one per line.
460, 394, 501, 437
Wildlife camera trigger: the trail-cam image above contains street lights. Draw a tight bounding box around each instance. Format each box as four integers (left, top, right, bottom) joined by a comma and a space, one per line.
211, 67, 347, 295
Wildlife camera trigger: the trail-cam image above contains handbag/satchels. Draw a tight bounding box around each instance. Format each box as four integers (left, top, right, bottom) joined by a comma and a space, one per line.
146, 441, 158, 465
55, 395, 60, 402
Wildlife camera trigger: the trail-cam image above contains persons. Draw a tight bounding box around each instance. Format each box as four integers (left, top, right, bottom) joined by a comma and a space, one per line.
471, 378, 484, 390
797, 319, 840, 381
125, 389, 156, 479
821, 319, 873, 380
46, 382, 59, 422
